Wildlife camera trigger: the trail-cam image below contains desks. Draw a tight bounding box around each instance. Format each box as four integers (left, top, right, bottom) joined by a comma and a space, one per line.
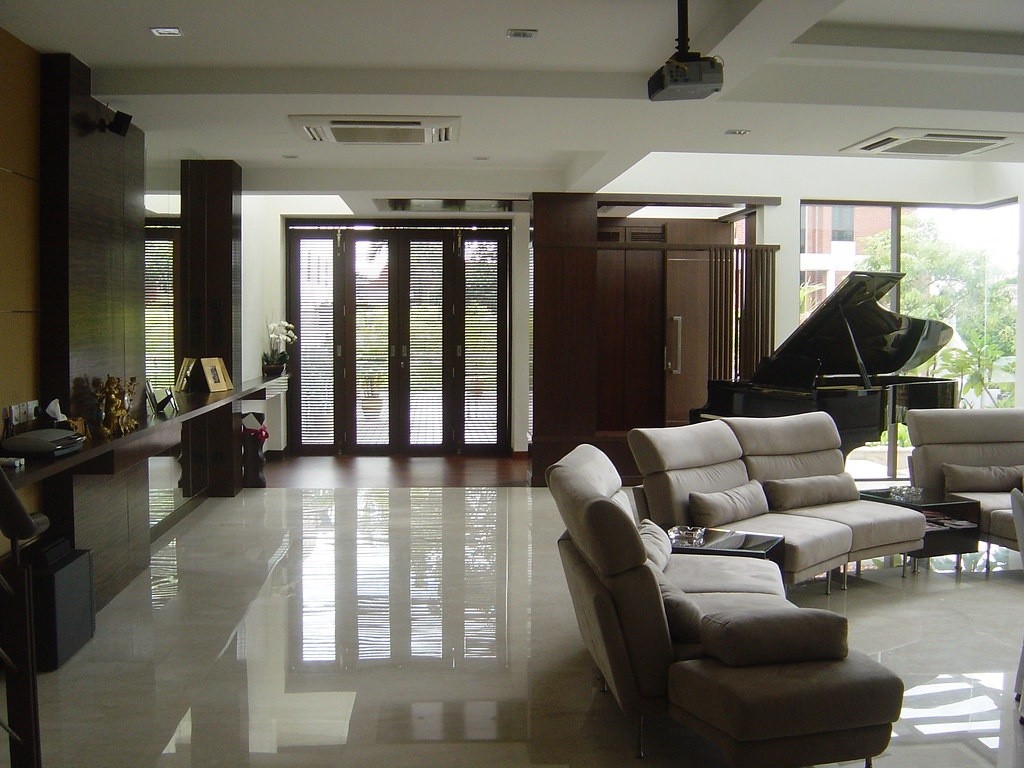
4, 373, 291, 491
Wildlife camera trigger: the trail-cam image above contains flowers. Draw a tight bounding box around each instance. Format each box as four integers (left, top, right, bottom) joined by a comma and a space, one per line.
261, 311, 298, 365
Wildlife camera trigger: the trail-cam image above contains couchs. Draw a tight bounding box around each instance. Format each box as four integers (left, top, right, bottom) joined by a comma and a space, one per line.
906, 408, 1024, 569
545, 443, 802, 759
626, 410, 926, 598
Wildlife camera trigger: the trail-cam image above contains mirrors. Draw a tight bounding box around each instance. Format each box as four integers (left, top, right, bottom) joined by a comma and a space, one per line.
143, 127, 211, 543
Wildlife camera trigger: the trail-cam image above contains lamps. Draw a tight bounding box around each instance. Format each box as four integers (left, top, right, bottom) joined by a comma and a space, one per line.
99, 110, 133, 138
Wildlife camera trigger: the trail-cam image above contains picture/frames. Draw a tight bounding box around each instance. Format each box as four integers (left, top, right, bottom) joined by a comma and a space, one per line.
173, 357, 196, 392
218, 358, 234, 390
200, 358, 228, 393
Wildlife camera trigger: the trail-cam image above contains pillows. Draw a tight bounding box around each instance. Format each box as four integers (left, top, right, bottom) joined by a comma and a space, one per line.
634, 518, 672, 571
698, 607, 848, 667
763, 471, 860, 511
688, 478, 770, 529
942, 463, 1024, 492
642, 557, 707, 642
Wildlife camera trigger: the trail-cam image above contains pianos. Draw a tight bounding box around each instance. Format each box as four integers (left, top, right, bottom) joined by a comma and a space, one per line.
688, 271, 959, 478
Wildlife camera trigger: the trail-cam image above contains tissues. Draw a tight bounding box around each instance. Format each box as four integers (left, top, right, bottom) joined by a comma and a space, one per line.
46, 398, 72, 430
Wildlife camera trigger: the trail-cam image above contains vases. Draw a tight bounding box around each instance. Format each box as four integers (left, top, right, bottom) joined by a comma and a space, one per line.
262, 363, 284, 378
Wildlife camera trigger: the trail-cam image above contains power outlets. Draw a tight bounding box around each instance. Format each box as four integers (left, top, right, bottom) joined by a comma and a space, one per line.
10, 400, 40, 426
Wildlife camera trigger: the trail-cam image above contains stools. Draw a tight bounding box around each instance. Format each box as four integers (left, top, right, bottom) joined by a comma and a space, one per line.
669, 648, 904, 768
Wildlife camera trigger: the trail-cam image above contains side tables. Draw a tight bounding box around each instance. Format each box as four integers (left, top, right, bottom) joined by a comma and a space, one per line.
855, 483, 982, 576
657, 522, 785, 588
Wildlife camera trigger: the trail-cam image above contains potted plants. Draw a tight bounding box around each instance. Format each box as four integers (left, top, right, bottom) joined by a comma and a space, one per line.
356, 369, 391, 418
